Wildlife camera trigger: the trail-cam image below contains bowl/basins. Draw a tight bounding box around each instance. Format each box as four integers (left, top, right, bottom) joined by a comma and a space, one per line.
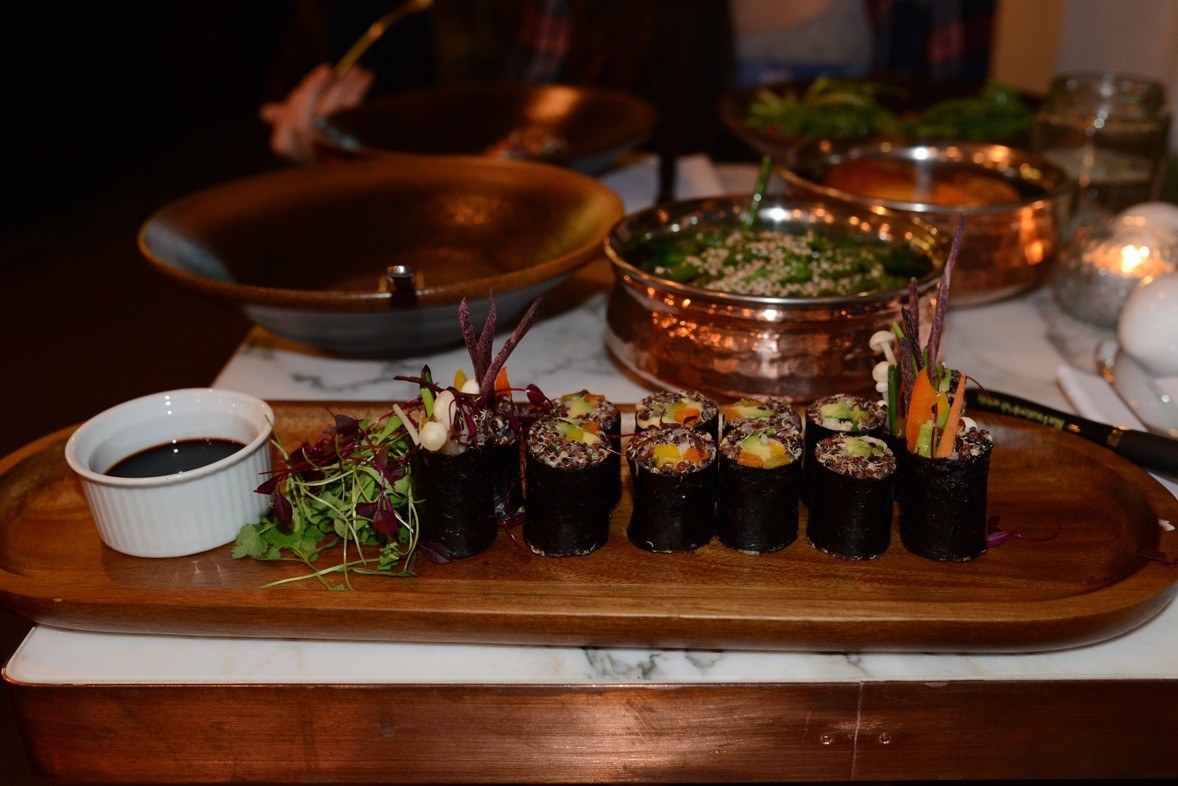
606, 58, 1170, 404
137, 155, 622, 357
62, 389, 277, 556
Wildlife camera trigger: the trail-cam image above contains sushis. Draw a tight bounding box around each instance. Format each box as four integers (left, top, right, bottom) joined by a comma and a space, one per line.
403, 365, 997, 561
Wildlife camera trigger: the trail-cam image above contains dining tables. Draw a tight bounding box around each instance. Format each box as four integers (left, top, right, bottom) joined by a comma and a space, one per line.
1, 149, 1178, 786
